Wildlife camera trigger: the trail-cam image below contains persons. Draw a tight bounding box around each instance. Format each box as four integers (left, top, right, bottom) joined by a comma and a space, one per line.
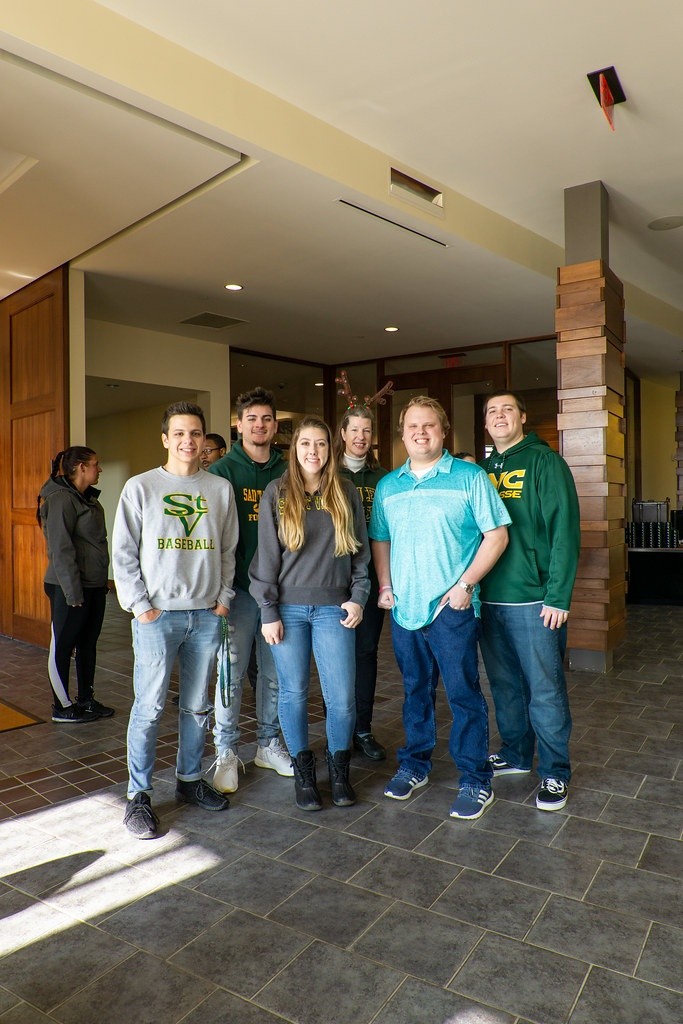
453, 452, 476, 463
475, 389, 580, 811
36, 446, 116, 723
368, 395, 513, 819
110, 400, 239, 839
171, 434, 257, 704
205, 387, 294, 792
322, 405, 387, 760
247, 413, 371, 809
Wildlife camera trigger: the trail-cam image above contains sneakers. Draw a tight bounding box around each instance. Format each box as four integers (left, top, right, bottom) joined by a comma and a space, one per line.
383, 770, 429, 800
536, 778, 568, 811
175, 778, 229, 811
489, 754, 531, 777
122, 792, 159, 839
254, 738, 295, 777
206, 748, 245, 793
449, 787, 494, 820
75, 696, 115, 717
52, 703, 101, 722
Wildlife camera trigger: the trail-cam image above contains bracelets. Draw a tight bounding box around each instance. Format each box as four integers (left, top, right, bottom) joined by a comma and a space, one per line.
379, 585, 392, 593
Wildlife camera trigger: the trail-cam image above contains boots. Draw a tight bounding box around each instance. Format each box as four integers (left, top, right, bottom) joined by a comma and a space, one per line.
326, 747, 356, 806
287, 750, 322, 811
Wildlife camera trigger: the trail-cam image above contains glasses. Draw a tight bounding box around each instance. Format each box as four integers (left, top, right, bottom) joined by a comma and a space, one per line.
200, 448, 220, 456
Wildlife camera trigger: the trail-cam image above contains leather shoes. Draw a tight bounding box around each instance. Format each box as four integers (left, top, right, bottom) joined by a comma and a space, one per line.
353, 733, 386, 760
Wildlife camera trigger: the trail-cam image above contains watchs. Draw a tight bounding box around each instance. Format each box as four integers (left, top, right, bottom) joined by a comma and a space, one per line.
457, 580, 476, 594
